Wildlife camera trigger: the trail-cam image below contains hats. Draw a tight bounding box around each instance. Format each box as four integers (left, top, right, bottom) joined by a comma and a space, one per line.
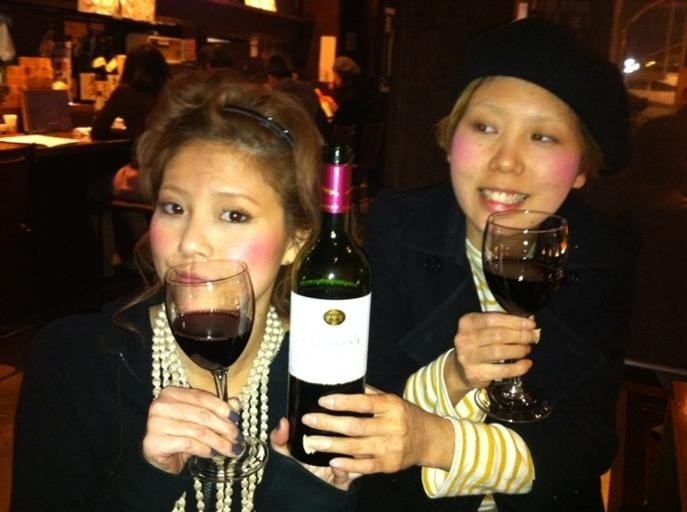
454, 16, 631, 184
335, 56, 363, 76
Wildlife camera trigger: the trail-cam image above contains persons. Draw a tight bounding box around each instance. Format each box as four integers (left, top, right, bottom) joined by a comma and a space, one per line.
265, 56, 332, 132
332, 56, 376, 121
199, 43, 232, 70
301, 16, 632, 512
90, 44, 168, 140
5, 66, 356, 512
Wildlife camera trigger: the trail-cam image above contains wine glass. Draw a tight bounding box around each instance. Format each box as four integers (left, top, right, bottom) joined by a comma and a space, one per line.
474, 209, 570, 424
163, 260, 268, 482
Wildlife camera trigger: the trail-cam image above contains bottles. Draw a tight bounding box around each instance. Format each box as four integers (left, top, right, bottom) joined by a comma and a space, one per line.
286, 141, 372, 467
77, 29, 120, 103
52, 33, 72, 78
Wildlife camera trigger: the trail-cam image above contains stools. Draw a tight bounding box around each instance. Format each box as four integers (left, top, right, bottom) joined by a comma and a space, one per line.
109, 201, 156, 294
601, 360, 687, 511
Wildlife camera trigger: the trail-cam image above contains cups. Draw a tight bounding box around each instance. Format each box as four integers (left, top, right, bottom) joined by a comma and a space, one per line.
2, 114, 18, 132
